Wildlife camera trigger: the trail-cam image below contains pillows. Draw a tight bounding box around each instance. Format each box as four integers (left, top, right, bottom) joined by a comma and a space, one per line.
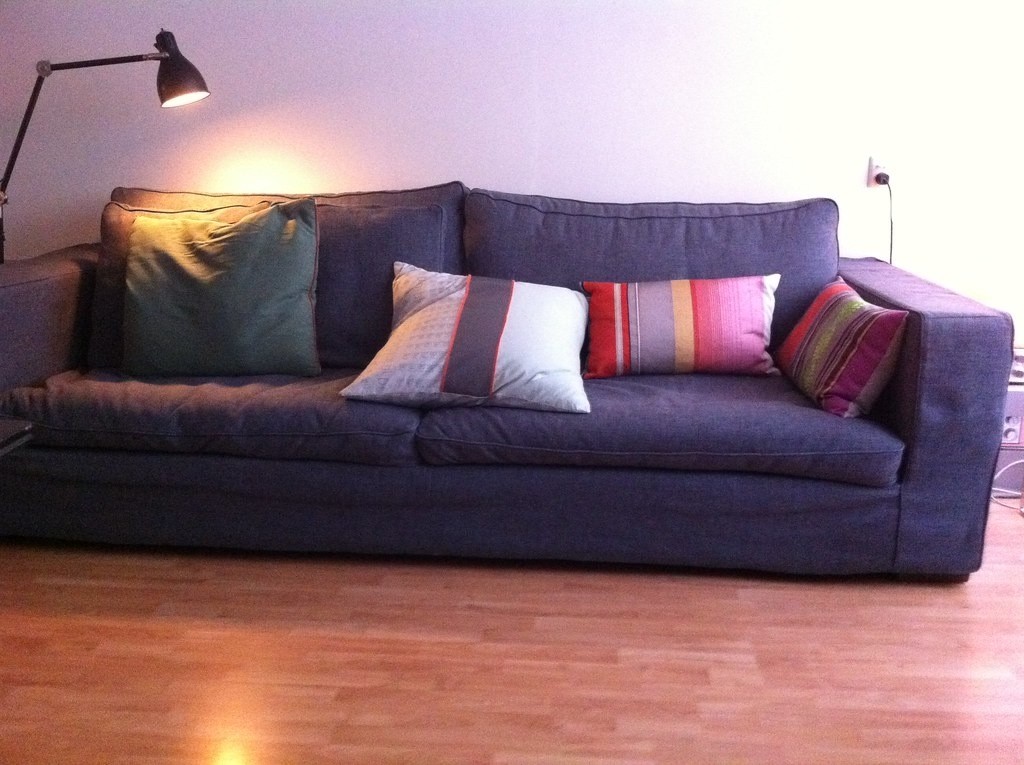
462, 187, 840, 354
578, 274, 784, 379
87, 199, 449, 371
771, 275, 908, 419
109, 180, 470, 277
339, 261, 592, 420
115, 195, 323, 379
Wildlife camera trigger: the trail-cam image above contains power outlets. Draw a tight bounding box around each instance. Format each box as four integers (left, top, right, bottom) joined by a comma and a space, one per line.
866, 156, 891, 188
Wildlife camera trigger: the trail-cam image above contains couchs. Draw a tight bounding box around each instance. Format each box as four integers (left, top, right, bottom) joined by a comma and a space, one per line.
1, 242, 1016, 590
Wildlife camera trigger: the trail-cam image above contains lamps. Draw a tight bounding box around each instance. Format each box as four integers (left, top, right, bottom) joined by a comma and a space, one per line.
0, 25, 210, 264
